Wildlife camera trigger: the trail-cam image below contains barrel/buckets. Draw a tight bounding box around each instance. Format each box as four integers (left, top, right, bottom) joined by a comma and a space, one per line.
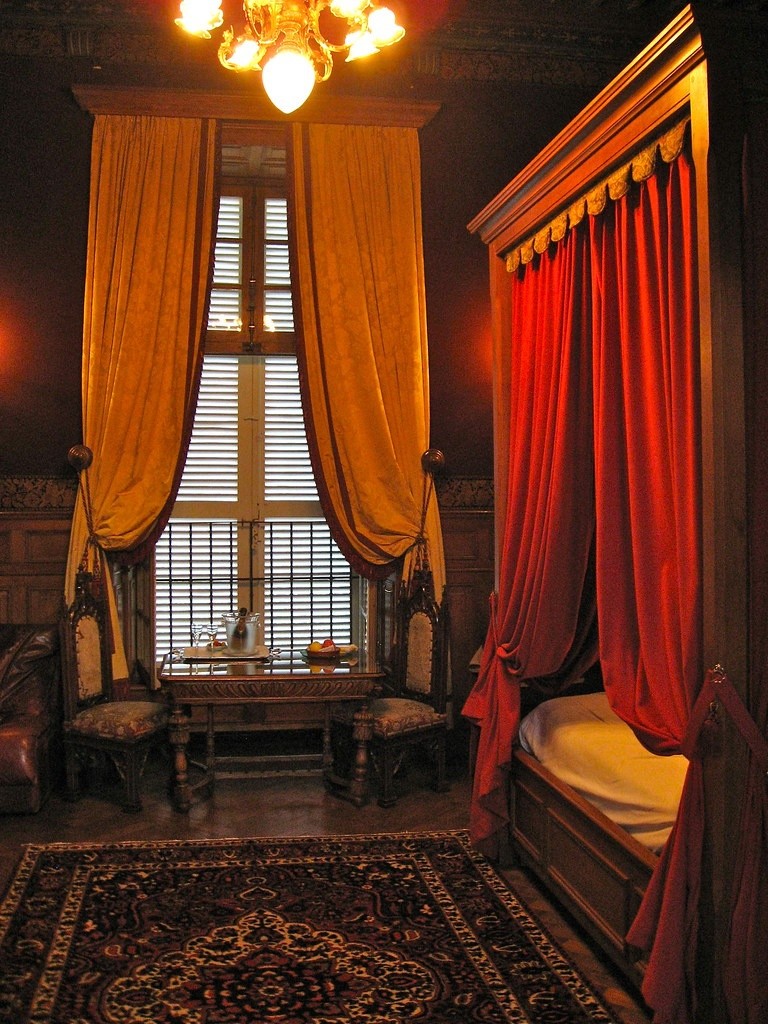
223, 610, 261, 655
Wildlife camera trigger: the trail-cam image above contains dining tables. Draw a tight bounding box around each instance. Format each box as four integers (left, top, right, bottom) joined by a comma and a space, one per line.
158, 649, 389, 816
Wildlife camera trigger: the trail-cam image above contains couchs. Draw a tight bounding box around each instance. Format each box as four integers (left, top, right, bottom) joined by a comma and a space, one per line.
0, 622, 62, 819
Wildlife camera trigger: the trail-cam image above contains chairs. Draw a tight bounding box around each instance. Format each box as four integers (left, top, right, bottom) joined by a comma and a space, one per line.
57, 564, 174, 815
323, 561, 454, 810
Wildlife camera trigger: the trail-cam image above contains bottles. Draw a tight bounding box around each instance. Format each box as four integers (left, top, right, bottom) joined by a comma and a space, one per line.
230, 607, 249, 651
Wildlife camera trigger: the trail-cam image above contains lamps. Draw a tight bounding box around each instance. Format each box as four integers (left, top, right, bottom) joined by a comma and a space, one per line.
172, 0, 406, 116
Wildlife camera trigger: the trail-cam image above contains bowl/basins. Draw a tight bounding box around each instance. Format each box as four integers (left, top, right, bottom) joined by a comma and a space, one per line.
306, 646, 340, 659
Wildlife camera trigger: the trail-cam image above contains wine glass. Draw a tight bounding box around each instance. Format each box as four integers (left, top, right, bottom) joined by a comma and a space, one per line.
207, 623, 219, 657
192, 621, 202, 656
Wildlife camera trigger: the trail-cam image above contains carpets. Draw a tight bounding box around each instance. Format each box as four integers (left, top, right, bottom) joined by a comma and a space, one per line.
0, 825, 654, 1024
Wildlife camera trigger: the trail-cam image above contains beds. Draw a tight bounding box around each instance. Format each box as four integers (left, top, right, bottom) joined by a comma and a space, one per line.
501, 683, 768, 1024
